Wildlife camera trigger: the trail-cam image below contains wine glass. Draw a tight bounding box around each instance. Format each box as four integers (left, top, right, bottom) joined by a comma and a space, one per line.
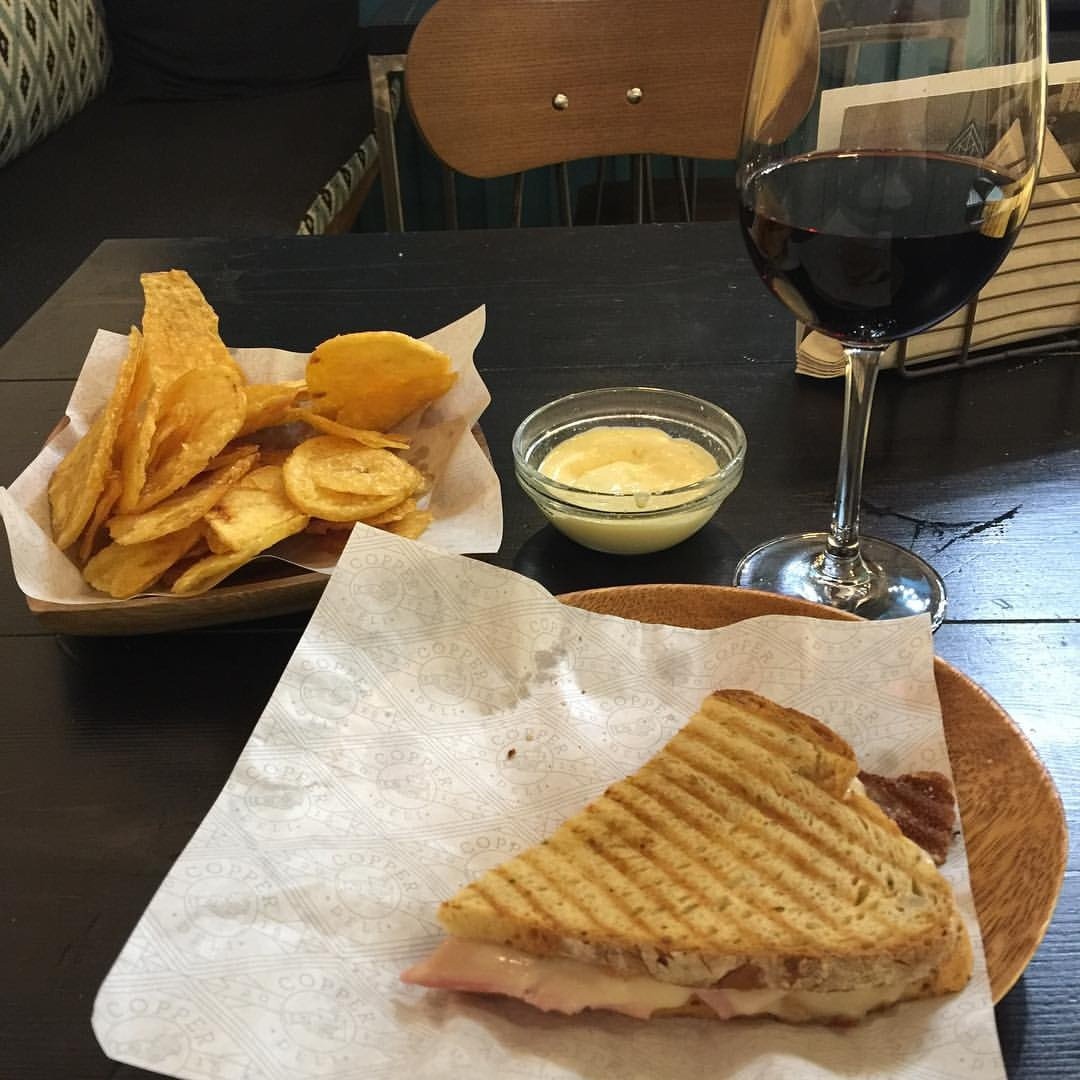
736, 1, 1053, 632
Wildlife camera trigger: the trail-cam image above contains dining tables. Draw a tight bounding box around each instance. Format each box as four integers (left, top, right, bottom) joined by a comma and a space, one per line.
0, 218, 1080, 1079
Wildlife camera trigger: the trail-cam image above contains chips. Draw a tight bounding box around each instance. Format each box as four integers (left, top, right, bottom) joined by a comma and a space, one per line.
46, 269, 458, 596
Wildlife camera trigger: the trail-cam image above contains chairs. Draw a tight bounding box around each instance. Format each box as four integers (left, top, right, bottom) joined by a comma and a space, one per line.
405, 0, 819, 229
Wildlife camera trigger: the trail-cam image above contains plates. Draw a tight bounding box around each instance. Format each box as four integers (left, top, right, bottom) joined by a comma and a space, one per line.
525, 585, 1066, 1015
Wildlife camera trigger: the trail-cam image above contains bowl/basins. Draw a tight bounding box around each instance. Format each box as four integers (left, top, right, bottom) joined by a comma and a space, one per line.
510, 383, 750, 551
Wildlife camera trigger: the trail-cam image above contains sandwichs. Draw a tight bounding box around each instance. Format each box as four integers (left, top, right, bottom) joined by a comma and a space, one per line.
394, 688, 975, 1024
